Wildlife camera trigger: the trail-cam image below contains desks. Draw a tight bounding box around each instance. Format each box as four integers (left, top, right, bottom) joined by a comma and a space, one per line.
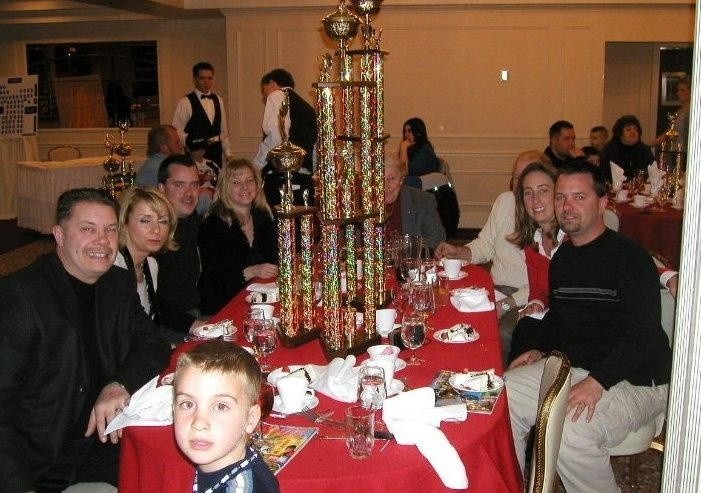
15, 154, 150, 234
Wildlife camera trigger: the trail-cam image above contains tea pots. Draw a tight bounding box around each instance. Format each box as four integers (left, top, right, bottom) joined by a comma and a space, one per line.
394, 231, 432, 279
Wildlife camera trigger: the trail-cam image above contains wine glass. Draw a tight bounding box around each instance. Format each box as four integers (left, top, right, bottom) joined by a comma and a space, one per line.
252, 381, 274, 450
255, 323, 277, 373
403, 313, 427, 365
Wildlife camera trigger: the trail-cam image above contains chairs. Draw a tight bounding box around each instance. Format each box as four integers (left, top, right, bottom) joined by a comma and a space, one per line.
604, 251, 678, 489
533, 346, 575, 492
48, 144, 82, 161
402, 171, 460, 240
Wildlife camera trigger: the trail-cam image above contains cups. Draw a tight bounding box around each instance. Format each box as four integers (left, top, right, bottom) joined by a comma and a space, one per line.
276, 377, 316, 410
366, 345, 402, 390
611, 170, 658, 208
345, 405, 375, 458
246, 257, 463, 337
358, 367, 389, 411
244, 318, 266, 341
213, 136, 219, 141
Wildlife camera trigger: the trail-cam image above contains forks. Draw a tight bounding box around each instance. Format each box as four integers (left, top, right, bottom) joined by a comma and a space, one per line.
185, 335, 237, 343
301, 406, 396, 439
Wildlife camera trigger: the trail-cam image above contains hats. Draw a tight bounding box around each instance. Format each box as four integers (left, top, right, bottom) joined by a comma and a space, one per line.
186, 133, 208, 152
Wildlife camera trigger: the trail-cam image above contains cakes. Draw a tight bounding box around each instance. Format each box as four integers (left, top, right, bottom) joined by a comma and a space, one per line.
284, 363, 317, 384
247, 289, 273, 303
201, 320, 234, 336
441, 323, 477, 342
459, 368, 497, 392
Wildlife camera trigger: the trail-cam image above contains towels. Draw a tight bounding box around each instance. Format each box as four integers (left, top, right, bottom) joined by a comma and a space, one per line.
301, 348, 405, 403
378, 385, 471, 490
101, 372, 176, 436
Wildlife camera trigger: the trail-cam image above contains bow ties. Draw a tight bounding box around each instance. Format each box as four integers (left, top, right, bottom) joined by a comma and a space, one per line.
201, 94, 214, 99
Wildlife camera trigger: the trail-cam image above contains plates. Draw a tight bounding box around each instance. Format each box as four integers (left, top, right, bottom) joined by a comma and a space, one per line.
386, 378, 406, 397
267, 364, 304, 387
194, 323, 240, 337
273, 393, 319, 414
438, 269, 469, 280
449, 370, 504, 391
432, 329, 480, 343
362, 357, 406, 372
